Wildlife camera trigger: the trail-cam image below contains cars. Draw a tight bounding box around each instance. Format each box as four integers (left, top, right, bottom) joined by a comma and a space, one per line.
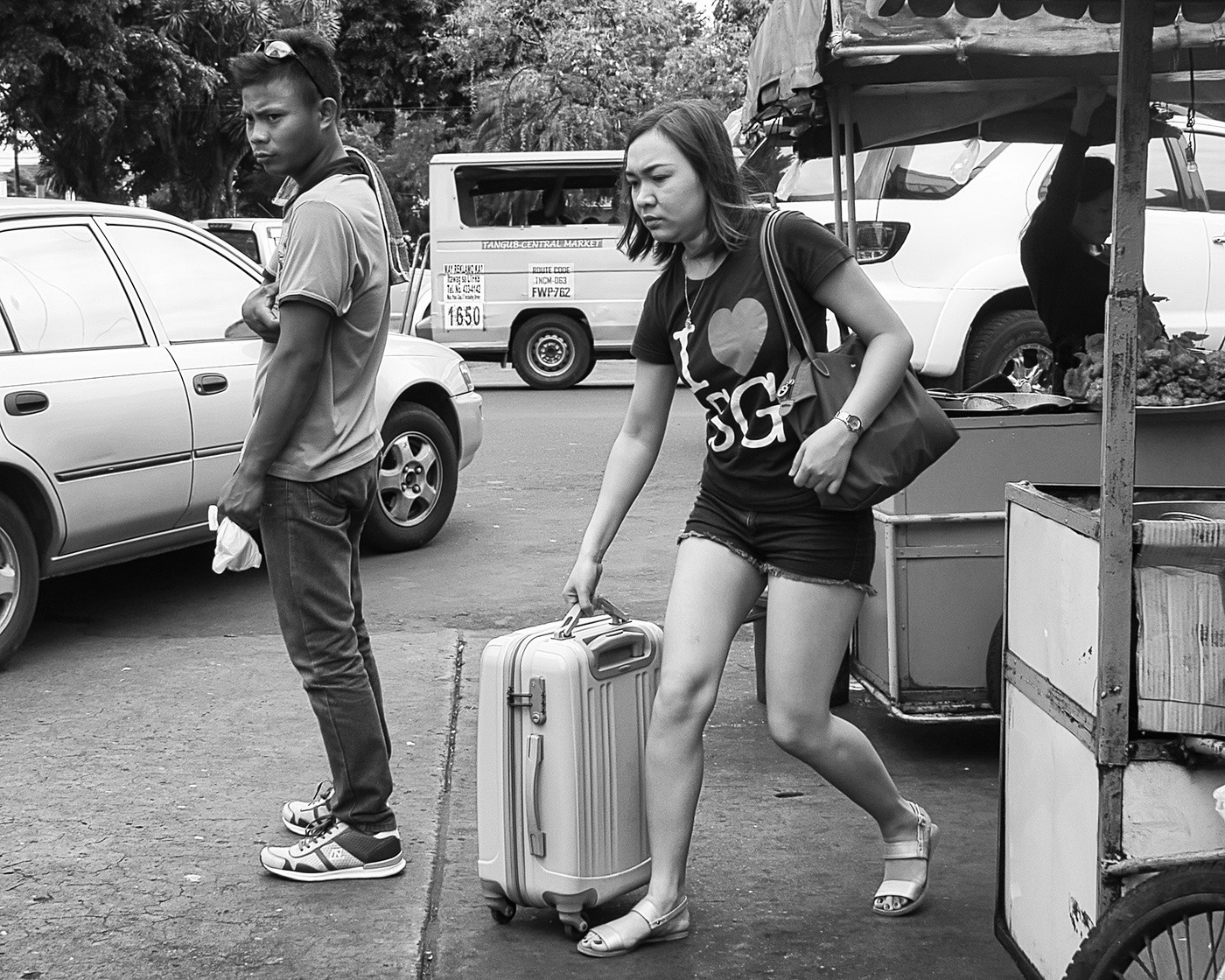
187, 216, 431, 340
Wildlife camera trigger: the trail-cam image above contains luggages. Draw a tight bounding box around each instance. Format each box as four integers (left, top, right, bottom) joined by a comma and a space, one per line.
476, 599, 666, 940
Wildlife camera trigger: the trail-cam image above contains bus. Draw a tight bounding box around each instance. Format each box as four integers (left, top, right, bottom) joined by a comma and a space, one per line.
413, 148, 679, 391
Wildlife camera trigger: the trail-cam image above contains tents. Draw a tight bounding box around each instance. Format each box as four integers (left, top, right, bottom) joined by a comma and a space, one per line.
744, 0, 1225, 315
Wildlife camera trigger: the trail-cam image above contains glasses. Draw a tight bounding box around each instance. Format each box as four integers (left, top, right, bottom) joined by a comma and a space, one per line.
253, 38, 324, 101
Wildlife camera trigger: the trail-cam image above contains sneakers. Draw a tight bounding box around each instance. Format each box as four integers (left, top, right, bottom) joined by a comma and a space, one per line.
282, 779, 343, 834
260, 814, 407, 882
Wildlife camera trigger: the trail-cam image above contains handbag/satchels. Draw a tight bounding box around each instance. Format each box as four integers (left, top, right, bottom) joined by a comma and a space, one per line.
760, 208, 960, 511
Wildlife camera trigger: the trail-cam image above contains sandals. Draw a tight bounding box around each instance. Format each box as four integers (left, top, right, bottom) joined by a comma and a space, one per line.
576, 896, 690, 957
874, 801, 940, 916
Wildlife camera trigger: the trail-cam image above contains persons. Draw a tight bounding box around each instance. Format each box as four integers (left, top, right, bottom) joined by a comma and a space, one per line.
527, 187, 575, 226
1017, 75, 1161, 392
228, 32, 409, 886
562, 99, 942, 957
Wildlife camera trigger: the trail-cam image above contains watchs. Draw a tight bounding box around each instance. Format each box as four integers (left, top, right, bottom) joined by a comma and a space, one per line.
834, 410, 865, 438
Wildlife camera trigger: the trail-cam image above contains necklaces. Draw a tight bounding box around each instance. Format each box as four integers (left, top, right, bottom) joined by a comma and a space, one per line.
682, 243, 718, 331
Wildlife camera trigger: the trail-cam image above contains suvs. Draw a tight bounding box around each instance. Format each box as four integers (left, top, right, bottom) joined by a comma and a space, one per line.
722, 99, 1224, 400
0, 193, 484, 673
